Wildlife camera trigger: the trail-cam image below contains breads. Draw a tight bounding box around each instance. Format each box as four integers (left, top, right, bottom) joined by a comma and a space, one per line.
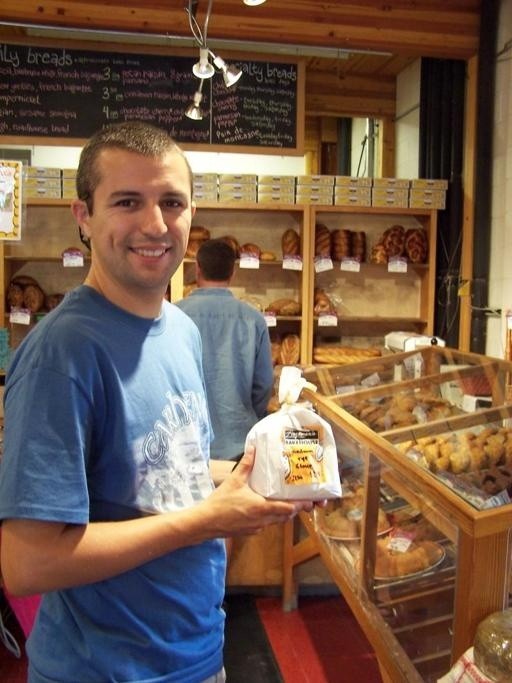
474, 605, 512, 682
270, 332, 300, 365
315, 288, 335, 316
185, 224, 429, 264
314, 345, 404, 365
316, 387, 512, 578
6, 275, 64, 312
264, 295, 302, 317
60, 246, 85, 258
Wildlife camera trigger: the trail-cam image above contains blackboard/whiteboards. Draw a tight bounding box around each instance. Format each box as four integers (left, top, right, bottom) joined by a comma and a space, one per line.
0, 36, 305, 156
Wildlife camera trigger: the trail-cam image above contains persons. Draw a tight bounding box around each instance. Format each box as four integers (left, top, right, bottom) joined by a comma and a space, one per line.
173, 240, 275, 558
0, 120, 329, 683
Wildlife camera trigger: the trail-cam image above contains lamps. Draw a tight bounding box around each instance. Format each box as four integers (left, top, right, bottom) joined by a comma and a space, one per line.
182, 1, 250, 119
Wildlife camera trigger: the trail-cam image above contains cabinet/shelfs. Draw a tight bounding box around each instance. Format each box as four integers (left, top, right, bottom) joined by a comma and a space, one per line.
180, 205, 305, 369
2, 205, 172, 357
308, 202, 436, 371
